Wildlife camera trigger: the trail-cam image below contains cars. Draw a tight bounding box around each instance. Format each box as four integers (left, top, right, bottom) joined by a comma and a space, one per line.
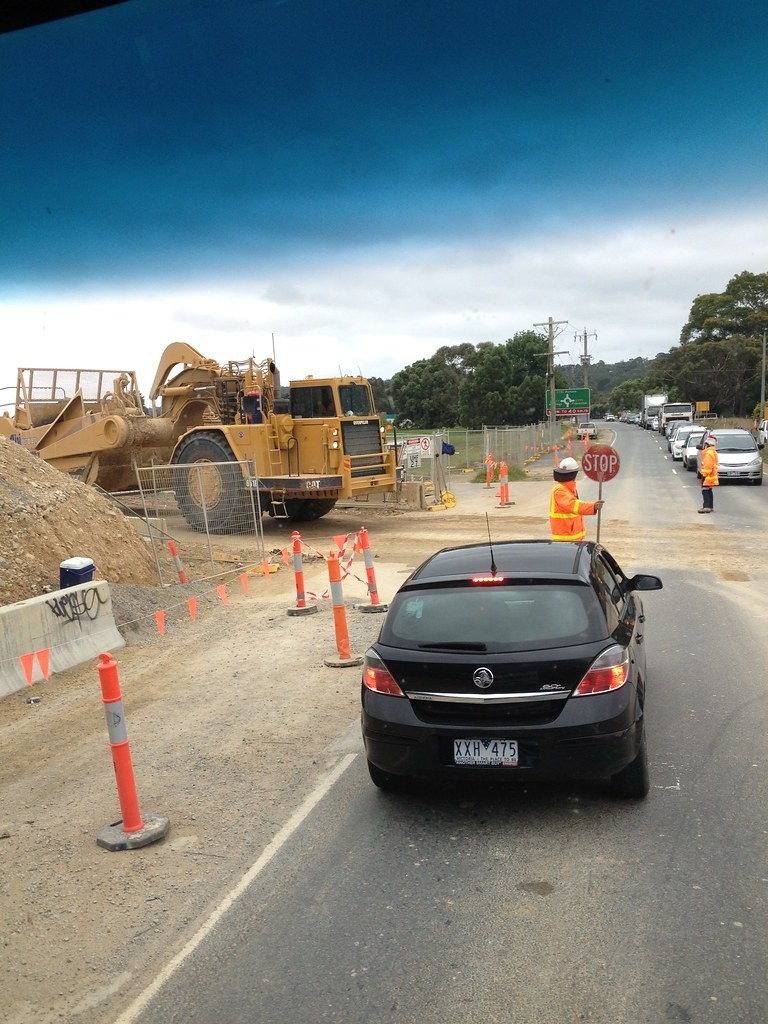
605, 393, 768, 484
360, 539, 664, 801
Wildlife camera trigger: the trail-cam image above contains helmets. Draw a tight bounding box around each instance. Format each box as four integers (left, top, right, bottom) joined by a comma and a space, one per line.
560, 457, 579, 470
705, 437, 716, 446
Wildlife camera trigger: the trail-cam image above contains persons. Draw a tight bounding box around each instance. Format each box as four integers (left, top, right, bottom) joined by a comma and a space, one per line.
549, 457, 606, 542
697, 436, 719, 513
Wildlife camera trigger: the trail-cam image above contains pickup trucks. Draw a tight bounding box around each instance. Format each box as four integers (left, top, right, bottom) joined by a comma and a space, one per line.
578, 423, 597, 439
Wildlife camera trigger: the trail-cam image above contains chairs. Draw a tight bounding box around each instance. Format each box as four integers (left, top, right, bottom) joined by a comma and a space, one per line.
243, 396, 263, 423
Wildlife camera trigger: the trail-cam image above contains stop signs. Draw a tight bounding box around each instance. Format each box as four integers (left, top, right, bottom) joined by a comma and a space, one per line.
582, 444, 621, 483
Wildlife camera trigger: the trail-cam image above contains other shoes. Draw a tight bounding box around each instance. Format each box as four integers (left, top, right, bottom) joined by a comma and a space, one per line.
698, 508, 711, 513
711, 508, 713, 512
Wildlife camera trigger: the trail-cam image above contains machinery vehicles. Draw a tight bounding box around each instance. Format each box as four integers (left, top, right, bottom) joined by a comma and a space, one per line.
0, 342, 397, 534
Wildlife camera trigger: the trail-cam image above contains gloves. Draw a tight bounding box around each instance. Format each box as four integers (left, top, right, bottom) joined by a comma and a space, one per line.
701, 476, 706, 485
